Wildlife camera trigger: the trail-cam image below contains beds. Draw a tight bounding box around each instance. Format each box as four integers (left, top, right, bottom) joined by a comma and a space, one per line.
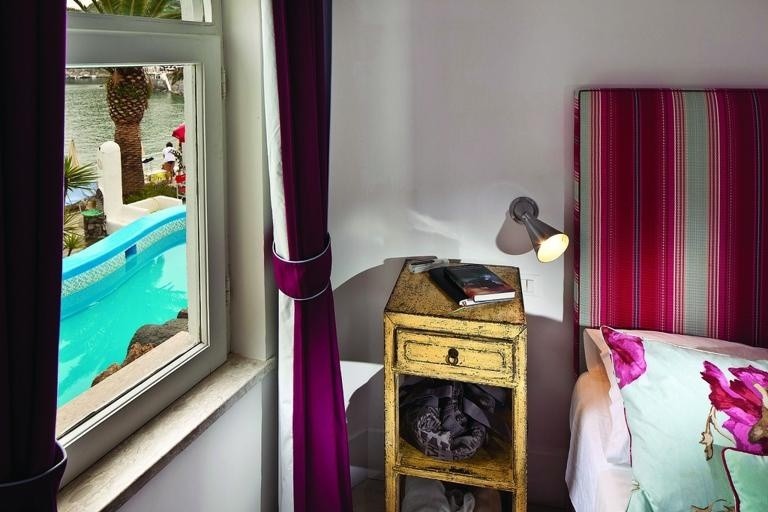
563, 86, 768, 511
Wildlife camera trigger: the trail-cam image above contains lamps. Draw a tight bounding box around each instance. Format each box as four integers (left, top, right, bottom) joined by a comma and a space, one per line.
507, 195, 571, 262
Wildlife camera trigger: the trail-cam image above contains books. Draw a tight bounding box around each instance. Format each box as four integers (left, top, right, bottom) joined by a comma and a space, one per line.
427, 267, 514, 307
443, 264, 516, 303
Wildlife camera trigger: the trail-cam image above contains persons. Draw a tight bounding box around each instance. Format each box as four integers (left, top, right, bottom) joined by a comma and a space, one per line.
163, 142, 177, 183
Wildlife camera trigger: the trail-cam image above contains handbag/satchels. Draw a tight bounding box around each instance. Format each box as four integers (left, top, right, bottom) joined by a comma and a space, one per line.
401, 380, 510, 462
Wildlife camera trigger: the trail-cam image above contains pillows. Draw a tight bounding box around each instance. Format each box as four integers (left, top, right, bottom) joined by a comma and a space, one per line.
581, 325, 767, 468
711, 444, 768, 510
600, 327, 768, 510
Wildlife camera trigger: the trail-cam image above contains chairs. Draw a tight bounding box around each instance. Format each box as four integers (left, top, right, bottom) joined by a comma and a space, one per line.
175, 170, 185, 199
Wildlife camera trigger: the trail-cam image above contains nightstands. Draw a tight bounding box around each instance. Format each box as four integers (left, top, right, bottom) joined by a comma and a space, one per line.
382, 257, 529, 510
